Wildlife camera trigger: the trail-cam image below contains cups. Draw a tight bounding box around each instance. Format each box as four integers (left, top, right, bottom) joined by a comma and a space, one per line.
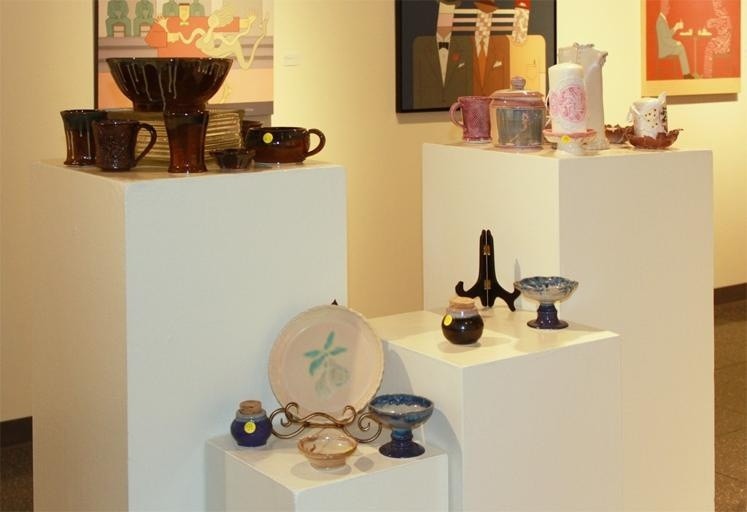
60, 107, 325, 171
449, 94, 497, 144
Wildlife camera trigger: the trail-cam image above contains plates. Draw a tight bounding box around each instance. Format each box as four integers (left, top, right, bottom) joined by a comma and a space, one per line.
267, 300, 387, 431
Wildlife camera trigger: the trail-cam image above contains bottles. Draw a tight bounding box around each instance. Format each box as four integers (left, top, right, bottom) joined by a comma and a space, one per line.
442, 297, 484, 345
231, 399, 273, 448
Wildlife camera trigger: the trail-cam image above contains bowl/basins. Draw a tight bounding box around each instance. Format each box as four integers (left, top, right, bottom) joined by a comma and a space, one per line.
109, 54, 235, 109
295, 436, 359, 469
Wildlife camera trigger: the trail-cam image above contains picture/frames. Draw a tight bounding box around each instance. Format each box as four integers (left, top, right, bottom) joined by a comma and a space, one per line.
395, 0, 557, 114
94, 0, 275, 117
642, 0, 746, 96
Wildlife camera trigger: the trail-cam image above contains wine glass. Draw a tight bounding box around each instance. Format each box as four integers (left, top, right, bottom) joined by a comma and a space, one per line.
367, 392, 429, 459
516, 274, 579, 332
